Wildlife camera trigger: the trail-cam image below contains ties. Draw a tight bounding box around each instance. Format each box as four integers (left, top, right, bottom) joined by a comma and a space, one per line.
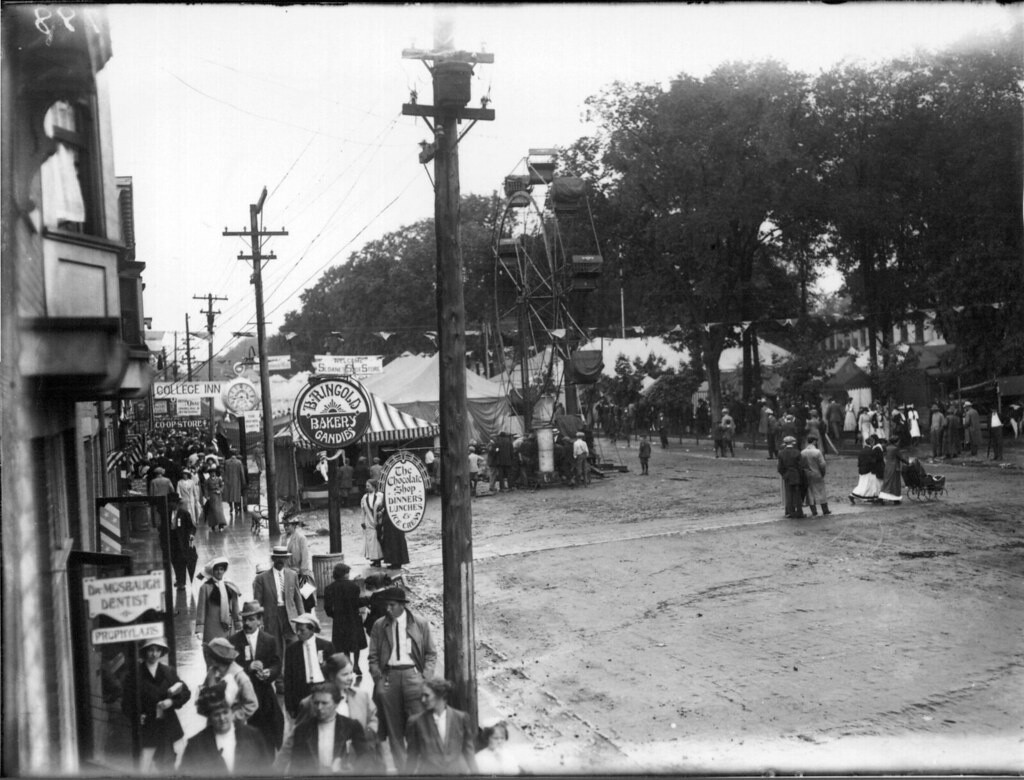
396, 619, 399, 660
279, 572, 284, 592
307, 644, 313, 680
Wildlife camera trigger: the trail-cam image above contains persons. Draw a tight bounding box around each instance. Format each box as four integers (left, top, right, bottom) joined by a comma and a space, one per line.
252, 444, 263, 472
361, 479, 411, 570
425, 404, 591, 496
122, 516, 478, 774
745, 394, 1004, 518
639, 436, 651, 475
132, 421, 246, 586
338, 456, 383, 506
593, 397, 736, 458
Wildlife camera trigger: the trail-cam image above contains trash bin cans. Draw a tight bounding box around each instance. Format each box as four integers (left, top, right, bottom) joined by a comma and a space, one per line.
311, 553, 344, 598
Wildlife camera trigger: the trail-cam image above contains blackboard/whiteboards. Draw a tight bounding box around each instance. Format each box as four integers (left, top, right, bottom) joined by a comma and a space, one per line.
246, 473, 260, 504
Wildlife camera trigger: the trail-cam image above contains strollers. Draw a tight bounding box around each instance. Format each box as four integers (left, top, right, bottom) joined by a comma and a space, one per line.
901, 460, 949, 504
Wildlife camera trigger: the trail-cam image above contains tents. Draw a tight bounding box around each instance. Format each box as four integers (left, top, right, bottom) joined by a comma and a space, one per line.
364, 352, 509, 443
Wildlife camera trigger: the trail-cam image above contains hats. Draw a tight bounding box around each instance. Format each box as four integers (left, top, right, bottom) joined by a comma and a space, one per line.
385, 588, 410, 603
198, 681, 228, 716
205, 556, 229, 576
270, 546, 292, 556
783, 436, 796, 443
238, 600, 264, 616
577, 432, 584, 437
291, 612, 321, 633
931, 405, 939, 410
202, 637, 239, 663
963, 401, 972, 407
280, 512, 299, 523
765, 408, 773, 414
138, 638, 170, 657
167, 492, 181, 501
552, 428, 559, 434
809, 410, 817, 416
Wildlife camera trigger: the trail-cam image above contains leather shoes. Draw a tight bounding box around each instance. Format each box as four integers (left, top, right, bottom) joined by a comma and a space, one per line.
385, 664, 416, 670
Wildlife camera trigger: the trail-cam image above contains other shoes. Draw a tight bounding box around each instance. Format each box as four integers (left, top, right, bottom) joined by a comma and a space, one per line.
353, 667, 362, 676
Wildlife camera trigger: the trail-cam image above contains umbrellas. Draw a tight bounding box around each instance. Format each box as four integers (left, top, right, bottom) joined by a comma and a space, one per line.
184, 538, 198, 583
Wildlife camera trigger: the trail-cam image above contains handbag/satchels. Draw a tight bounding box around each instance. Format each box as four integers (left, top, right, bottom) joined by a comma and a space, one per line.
300, 576, 316, 600
167, 681, 183, 697
